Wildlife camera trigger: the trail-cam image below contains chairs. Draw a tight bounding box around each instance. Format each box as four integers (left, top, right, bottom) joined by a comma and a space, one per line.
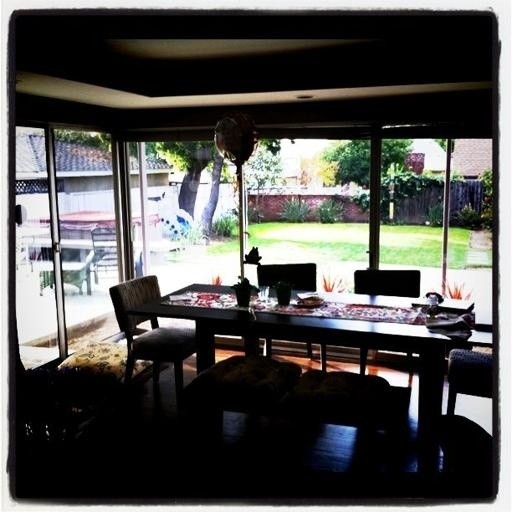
280, 369, 390, 427
91, 227, 121, 284
110, 276, 199, 416
353, 269, 420, 385
447, 345, 495, 418
256, 263, 329, 373
177, 352, 302, 416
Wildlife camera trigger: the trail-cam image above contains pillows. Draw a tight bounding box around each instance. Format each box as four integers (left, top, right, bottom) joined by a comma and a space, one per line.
58, 342, 130, 387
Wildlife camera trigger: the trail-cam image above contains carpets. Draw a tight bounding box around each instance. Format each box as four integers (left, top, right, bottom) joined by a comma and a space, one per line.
140, 366, 199, 415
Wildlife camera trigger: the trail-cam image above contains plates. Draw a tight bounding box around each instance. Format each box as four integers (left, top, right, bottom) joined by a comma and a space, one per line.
255, 299, 273, 305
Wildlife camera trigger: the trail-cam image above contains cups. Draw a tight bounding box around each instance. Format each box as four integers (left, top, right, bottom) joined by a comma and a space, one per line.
256, 287, 271, 302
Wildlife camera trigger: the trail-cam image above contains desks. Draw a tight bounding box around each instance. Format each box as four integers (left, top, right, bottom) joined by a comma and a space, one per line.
39, 260, 88, 296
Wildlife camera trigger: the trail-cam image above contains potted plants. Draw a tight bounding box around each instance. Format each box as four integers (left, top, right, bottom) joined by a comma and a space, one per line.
232, 275, 253, 307
